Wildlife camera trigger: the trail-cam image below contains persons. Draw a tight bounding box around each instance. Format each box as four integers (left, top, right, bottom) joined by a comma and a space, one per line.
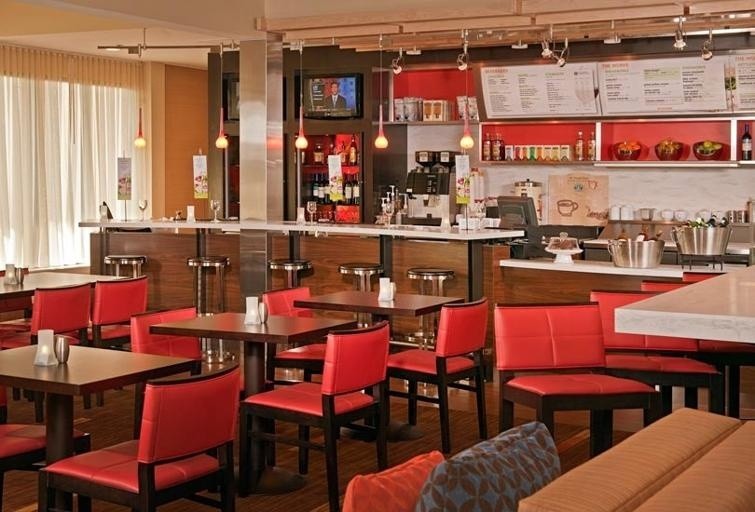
325, 82, 346, 108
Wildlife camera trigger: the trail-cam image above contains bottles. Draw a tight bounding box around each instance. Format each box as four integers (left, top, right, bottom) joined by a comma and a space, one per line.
345, 173, 352, 204
350, 136, 357, 165
319, 172, 324, 203
394, 97, 423, 122
499, 133, 505, 160
457, 96, 467, 119
326, 142, 334, 164
468, 97, 479, 120
491, 134, 494, 141
586, 132, 596, 160
338, 141, 349, 165
313, 172, 319, 203
575, 131, 586, 160
492, 133, 501, 160
476, 171, 485, 200
355, 173, 360, 204
325, 170, 330, 204
424, 101, 451, 122
742, 124, 752, 159
314, 143, 324, 164
483, 133, 491, 160
352, 175, 356, 204
471, 167, 479, 200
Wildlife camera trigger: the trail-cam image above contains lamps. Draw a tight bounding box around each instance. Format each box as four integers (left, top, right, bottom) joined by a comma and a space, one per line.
456, 29, 470, 71
460, 69, 474, 150
134, 45, 146, 149
295, 40, 308, 149
390, 46, 403, 74
541, 24, 569, 66
374, 34, 388, 149
701, 28, 713, 61
216, 44, 229, 148
673, 16, 686, 50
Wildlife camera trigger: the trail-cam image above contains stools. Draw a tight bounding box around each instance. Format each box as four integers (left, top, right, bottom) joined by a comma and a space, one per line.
187, 257, 231, 362
640, 280, 755, 426
407, 267, 455, 345
104, 256, 147, 348
268, 260, 313, 349
339, 263, 386, 327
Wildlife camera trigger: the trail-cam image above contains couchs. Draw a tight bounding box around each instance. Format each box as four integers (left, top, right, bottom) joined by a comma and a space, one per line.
520, 409, 755, 512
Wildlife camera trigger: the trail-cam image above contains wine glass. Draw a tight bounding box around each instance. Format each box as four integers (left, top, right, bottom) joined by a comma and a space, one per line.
474, 204, 486, 231
307, 202, 316, 224
138, 200, 147, 221
384, 203, 394, 230
210, 200, 221, 222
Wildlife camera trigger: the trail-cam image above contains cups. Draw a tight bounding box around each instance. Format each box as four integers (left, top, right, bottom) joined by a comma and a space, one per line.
609, 204, 634, 220
557, 200, 578, 217
675, 211, 689, 221
656, 210, 673, 221
640, 207, 657, 221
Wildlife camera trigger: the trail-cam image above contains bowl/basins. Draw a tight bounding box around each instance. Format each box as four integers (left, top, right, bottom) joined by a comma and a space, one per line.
670, 227, 733, 256
608, 240, 665, 268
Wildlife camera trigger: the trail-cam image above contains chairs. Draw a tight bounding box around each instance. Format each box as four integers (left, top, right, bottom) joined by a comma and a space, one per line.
37, 363, 240, 512
0, 283, 91, 422
130, 306, 276, 466
589, 289, 723, 458
0, 383, 93, 512
387, 297, 489, 453
494, 302, 661, 440
263, 286, 326, 390
62, 275, 149, 406
0, 290, 34, 332
239, 321, 390, 512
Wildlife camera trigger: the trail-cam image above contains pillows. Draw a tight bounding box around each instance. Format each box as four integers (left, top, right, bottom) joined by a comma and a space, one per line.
412, 421, 561, 512
345, 451, 444, 512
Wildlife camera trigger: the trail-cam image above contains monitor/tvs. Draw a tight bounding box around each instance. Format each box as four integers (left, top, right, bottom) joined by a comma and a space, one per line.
498, 197, 538, 227
293, 72, 364, 120
222, 76, 287, 121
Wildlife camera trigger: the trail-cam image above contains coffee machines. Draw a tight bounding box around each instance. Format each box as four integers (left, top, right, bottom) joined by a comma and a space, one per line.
402, 151, 467, 226
